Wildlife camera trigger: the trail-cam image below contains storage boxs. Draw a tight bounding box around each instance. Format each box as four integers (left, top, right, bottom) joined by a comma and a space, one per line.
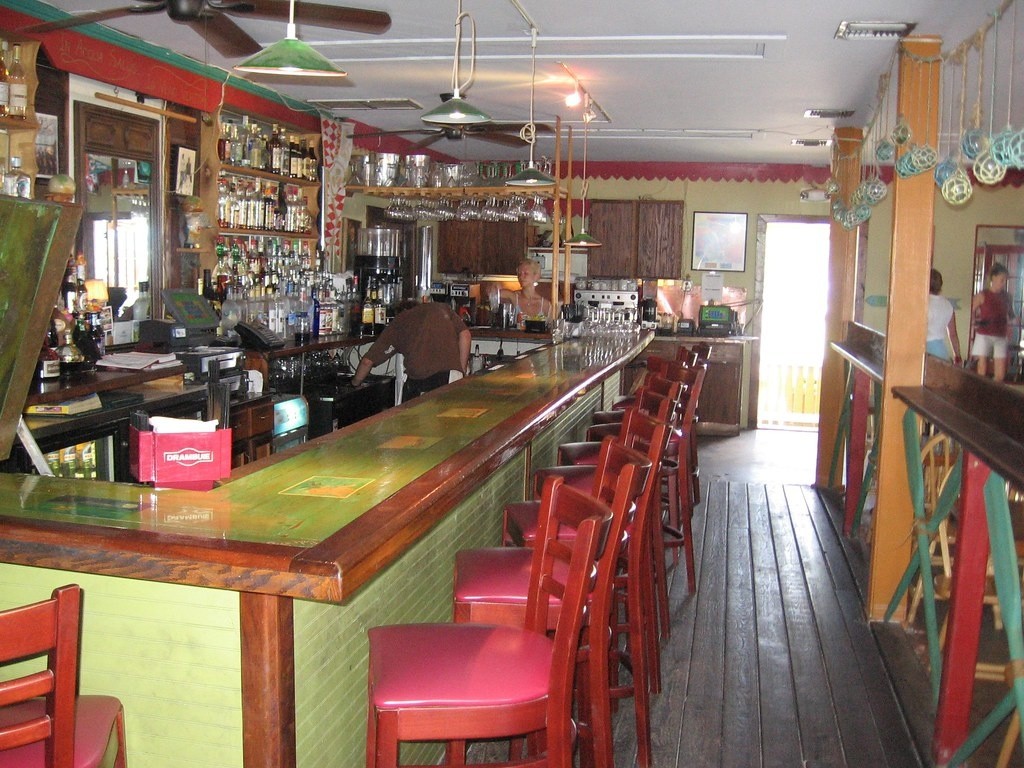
130, 426, 233, 492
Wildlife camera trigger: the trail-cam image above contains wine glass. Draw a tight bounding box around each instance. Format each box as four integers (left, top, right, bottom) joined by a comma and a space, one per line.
385, 156, 565, 226
580, 305, 642, 335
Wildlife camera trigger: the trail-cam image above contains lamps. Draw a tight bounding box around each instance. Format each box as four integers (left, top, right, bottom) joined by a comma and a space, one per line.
420, 1, 492, 124
232, 0, 348, 78
505, 28, 556, 186
563, 94, 602, 246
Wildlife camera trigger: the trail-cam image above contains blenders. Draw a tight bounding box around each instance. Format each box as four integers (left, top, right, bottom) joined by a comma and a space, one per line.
559, 304, 589, 339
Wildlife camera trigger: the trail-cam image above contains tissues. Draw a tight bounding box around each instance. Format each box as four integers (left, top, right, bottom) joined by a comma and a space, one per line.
147, 415, 232, 484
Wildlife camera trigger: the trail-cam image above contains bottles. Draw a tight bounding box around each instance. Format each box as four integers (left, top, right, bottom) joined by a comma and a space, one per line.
202, 115, 387, 345
638, 297, 658, 330
0, 37, 34, 200
39, 250, 105, 380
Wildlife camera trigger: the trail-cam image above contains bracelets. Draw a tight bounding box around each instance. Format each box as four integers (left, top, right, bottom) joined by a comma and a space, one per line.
350, 381, 361, 389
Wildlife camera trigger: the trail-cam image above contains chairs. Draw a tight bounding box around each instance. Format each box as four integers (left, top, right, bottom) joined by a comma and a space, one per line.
863, 382, 1024, 768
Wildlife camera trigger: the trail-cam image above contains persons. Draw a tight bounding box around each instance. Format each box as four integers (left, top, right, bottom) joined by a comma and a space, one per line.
486, 259, 553, 317
972, 262, 1020, 382
927, 269, 963, 366
346, 300, 471, 403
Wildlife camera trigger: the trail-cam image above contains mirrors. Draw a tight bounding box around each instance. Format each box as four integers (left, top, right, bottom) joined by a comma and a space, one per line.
74, 100, 163, 354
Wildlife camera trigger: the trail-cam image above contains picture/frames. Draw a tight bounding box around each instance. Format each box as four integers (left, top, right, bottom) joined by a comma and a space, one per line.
690, 211, 749, 272
171, 143, 198, 197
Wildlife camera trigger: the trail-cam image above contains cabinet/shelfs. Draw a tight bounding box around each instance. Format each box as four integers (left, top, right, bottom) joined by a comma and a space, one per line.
229, 331, 376, 468
641, 335, 758, 436
0, 29, 40, 195
277, 372, 396, 438
198, 106, 323, 311
438, 201, 527, 277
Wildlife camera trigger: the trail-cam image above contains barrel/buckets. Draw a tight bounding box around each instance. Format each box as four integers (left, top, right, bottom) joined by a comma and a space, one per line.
356, 225, 398, 257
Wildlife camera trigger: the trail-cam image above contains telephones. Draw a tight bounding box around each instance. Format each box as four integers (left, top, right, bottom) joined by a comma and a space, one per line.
233, 320, 285, 352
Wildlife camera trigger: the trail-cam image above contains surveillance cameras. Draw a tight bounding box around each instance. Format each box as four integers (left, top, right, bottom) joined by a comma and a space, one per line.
440, 92, 467, 103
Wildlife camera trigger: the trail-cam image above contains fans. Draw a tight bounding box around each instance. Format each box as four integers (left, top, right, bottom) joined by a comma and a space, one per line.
16, 0, 393, 58
347, 92, 555, 150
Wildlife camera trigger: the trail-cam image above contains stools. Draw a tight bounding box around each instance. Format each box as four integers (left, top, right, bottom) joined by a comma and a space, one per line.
1, 584, 128, 768
365, 342, 713, 768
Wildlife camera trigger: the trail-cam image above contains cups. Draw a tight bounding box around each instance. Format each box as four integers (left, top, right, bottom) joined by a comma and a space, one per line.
352, 152, 463, 187
575, 277, 639, 292
132, 281, 152, 321
551, 327, 564, 344
498, 303, 512, 329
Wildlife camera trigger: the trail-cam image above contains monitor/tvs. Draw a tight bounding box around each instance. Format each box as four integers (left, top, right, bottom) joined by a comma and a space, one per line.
162, 288, 220, 330
700, 306, 731, 324
